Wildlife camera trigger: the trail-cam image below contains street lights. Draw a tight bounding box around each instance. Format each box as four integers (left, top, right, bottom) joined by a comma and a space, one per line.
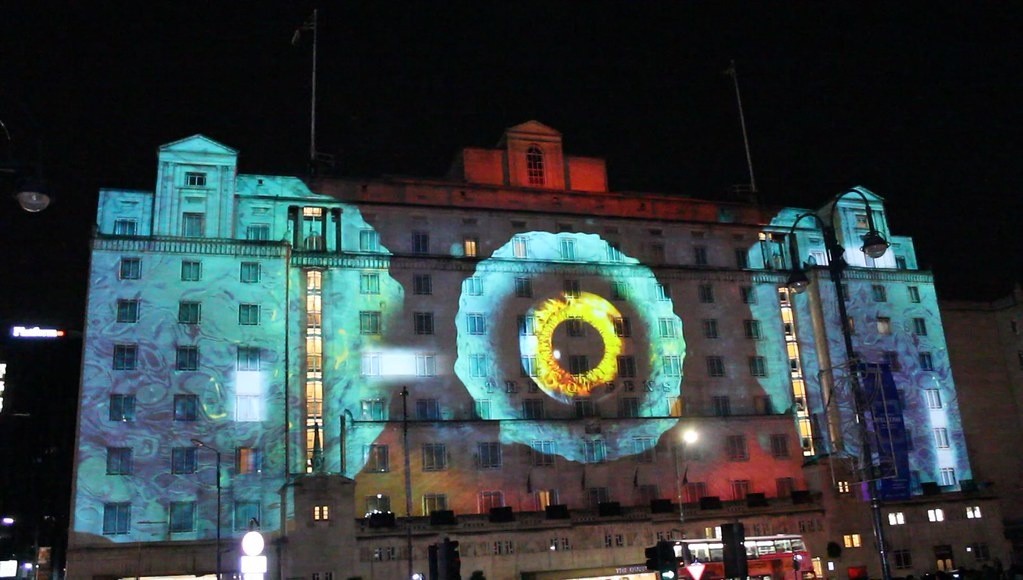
674, 428, 698, 539
400, 385, 414, 580
240, 532, 267, 580
786, 189, 892, 580
3, 511, 36, 580
190, 439, 220, 580
339, 409, 356, 475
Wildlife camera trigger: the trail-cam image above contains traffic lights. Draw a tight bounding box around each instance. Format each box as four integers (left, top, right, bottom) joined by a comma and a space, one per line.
645, 542, 662, 571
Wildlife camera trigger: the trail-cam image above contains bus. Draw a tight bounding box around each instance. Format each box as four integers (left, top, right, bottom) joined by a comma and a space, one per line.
656, 534, 817, 580
